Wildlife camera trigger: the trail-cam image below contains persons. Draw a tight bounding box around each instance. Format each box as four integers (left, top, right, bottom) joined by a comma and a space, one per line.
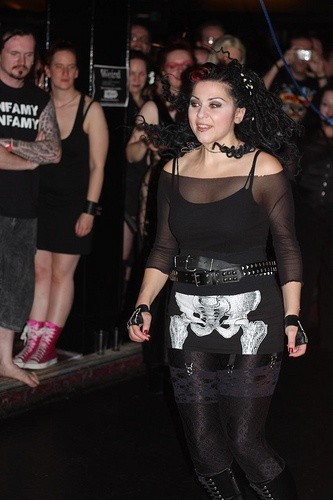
11, 39, 109, 370
0, 25, 62, 388
126, 58, 309, 500
122, 22, 333, 395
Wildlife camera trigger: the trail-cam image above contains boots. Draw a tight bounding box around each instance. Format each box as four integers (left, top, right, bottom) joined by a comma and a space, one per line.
195, 455, 248, 500
246, 458, 297, 500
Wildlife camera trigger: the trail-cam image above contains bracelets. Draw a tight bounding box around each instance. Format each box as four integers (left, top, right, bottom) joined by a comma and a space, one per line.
81, 199, 98, 216
8, 137, 14, 152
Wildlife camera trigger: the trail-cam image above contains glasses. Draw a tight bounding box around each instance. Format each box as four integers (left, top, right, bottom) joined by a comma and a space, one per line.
130, 70, 148, 81
130, 33, 150, 45
204, 36, 217, 46
165, 61, 190, 75
50, 63, 78, 72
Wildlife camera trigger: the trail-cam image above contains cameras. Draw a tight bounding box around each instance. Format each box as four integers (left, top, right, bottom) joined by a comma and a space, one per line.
297, 49, 312, 61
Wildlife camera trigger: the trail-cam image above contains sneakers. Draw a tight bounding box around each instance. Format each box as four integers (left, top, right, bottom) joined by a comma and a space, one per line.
13, 319, 44, 368
23, 321, 63, 369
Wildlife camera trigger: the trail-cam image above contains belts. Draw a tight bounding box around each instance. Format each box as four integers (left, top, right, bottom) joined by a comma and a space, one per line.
169, 255, 278, 287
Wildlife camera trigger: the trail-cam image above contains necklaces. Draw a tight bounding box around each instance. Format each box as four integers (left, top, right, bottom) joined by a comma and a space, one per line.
200, 140, 241, 154
